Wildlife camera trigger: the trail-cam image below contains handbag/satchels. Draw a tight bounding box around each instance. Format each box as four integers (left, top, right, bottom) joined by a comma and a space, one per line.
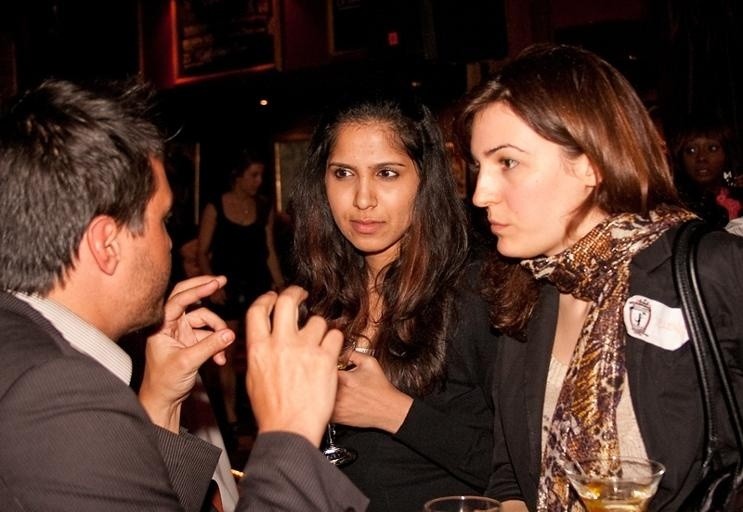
665, 442, 742, 512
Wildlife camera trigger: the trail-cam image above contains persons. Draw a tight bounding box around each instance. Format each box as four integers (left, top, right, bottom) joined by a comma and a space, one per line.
170, 150, 286, 452
3, 81, 370, 512
458, 42, 741, 512
288, 84, 500, 511
671, 111, 740, 228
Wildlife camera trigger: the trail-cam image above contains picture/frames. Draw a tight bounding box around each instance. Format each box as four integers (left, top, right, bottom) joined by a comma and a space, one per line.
10, 1, 437, 102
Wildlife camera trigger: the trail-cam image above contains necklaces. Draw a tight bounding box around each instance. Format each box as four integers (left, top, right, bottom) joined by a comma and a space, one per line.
239, 204, 249, 216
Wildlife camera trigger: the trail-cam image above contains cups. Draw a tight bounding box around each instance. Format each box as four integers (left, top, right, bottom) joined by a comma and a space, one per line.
422, 493, 501, 512
565, 456, 666, 512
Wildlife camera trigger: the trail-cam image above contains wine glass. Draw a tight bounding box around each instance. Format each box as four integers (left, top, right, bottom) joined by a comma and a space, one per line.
324, 343, 360, 467
